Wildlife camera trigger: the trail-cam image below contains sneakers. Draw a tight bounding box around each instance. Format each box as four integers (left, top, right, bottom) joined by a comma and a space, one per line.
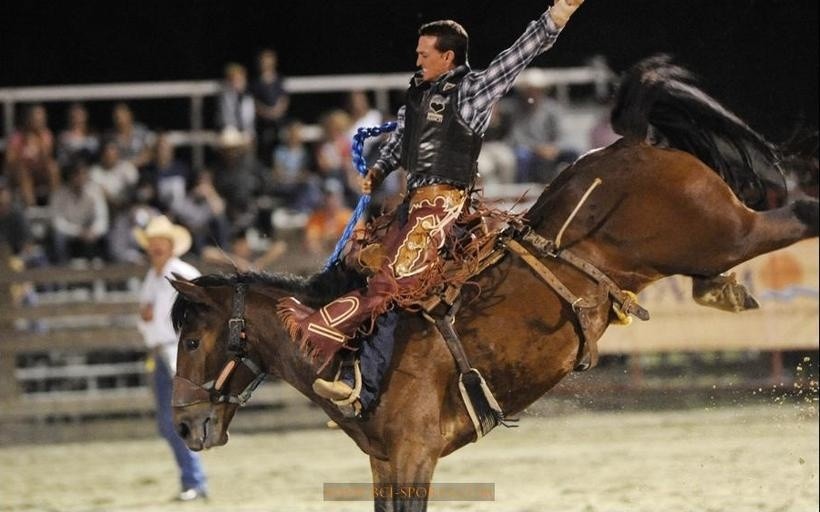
311, 378, 363, 416
178, 481, 210, 500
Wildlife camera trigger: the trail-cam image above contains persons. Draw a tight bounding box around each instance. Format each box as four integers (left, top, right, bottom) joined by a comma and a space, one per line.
0, 0, 623, 503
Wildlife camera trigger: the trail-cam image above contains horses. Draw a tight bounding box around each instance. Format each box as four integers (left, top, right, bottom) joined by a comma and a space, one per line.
164, 53, 820, 512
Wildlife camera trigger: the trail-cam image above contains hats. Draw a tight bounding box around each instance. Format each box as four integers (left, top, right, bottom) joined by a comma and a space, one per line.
131, 214, 193, 257
